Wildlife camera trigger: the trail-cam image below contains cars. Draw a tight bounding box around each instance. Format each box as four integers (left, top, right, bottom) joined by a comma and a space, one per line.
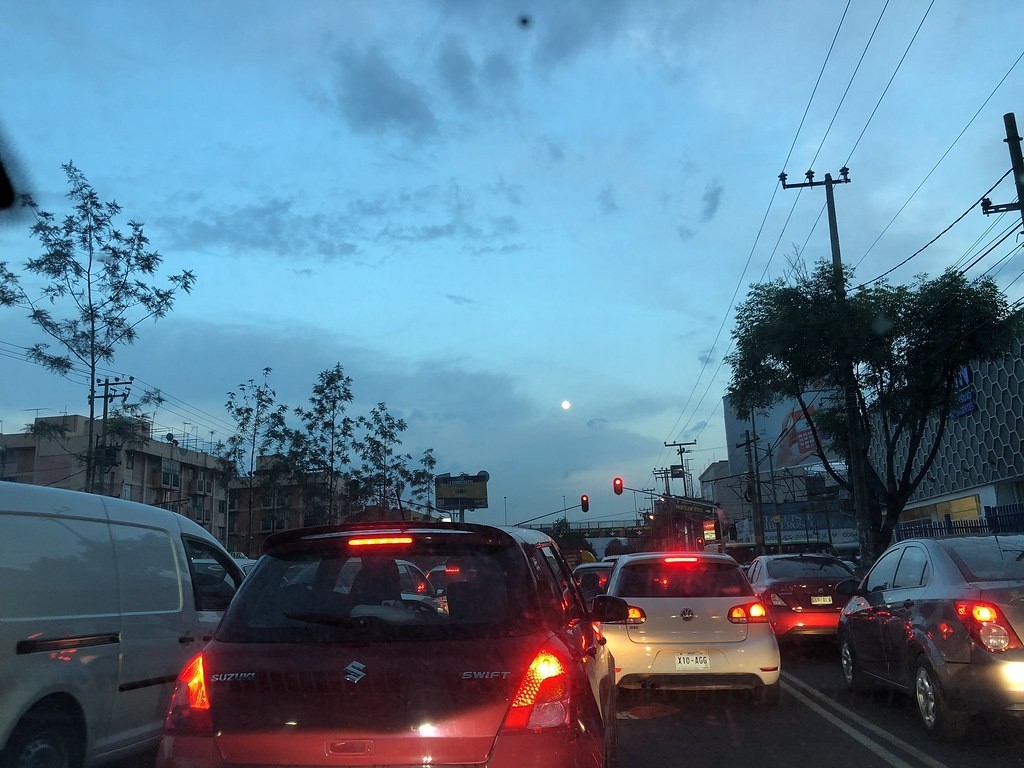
156, 521, 631, 768
560, 548, 619, 618
602, 550, 784, 706
286, 557, 446, 614
744, 553, 859, 649
835, 536, 1024, 741
190, 550, 259, 590
414, 564, 477, 602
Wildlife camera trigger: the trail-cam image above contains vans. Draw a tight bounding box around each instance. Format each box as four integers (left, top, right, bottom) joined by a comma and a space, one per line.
0, 476, 249, 768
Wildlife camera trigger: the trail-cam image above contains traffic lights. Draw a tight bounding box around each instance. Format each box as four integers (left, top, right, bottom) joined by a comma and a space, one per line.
581, 495, 589, 512
613, 477, 623, 495
696, 537, 705, 552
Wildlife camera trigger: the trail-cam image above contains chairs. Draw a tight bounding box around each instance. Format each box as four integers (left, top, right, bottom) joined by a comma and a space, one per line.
345, 557, 403, 606
579, 573, 604, 599
774, 564, 794, 578
446, 557, 505, 615
682, 568, 724, 596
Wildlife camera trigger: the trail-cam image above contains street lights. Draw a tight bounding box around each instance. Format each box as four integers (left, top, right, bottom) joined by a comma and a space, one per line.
562, 495, 568, 520
504, 495, 508, 525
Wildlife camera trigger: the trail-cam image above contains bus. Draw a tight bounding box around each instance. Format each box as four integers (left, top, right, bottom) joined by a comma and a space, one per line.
704, 539, 838, 577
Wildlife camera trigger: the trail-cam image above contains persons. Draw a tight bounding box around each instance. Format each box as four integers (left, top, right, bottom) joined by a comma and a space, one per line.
776, 408, 815, 470
